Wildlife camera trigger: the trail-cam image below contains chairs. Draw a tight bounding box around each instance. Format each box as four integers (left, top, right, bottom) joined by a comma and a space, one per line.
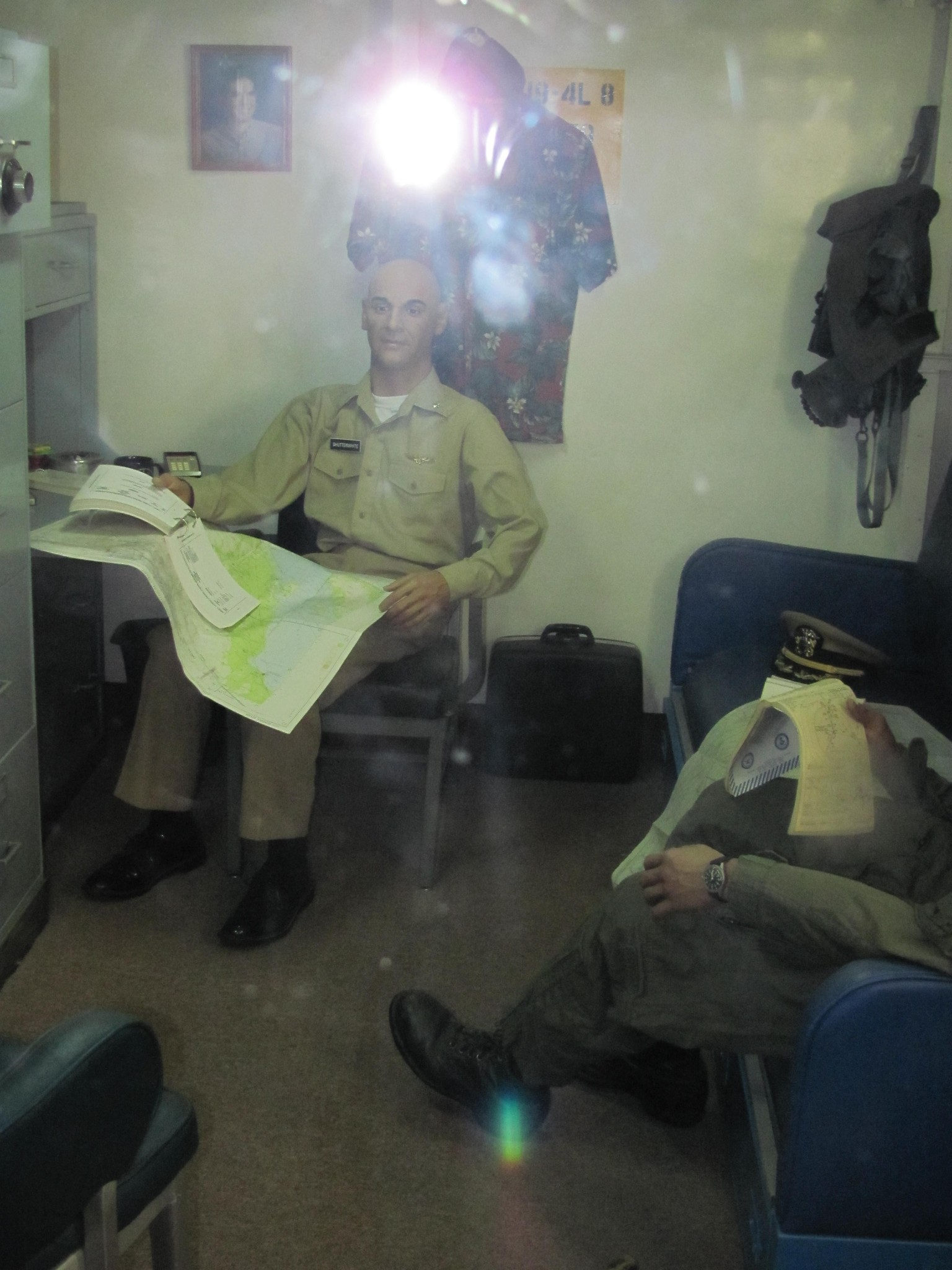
0, 1005, 203, 1270
317, 533, 493, 895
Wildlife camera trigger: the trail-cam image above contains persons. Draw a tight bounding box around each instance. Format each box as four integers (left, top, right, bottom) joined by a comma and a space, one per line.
80, 255, 548, 947
385, 679, 952, 1138
199, 68, 281, 168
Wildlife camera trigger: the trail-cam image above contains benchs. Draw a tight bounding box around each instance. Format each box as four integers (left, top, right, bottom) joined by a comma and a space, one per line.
664, 468, 951, 1269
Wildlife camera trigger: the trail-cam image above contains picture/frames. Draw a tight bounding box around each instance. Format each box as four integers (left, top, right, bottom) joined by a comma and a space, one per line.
188, 42, 295, 175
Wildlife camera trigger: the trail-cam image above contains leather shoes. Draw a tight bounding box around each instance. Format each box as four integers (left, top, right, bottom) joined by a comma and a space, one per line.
572, 1023, 707, 1128
386, 986, 550, 1145
81, 825, 202, 900
212, 842, 317, 950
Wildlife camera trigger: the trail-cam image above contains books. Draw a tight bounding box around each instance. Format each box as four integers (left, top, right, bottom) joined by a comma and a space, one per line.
68, 465, 262, 629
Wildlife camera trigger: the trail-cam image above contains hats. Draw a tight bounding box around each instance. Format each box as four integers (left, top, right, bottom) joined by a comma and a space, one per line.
775, 611, 890, 684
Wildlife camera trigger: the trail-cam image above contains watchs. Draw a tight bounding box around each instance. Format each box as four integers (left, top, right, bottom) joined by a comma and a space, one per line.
704, 856, 733, 904
